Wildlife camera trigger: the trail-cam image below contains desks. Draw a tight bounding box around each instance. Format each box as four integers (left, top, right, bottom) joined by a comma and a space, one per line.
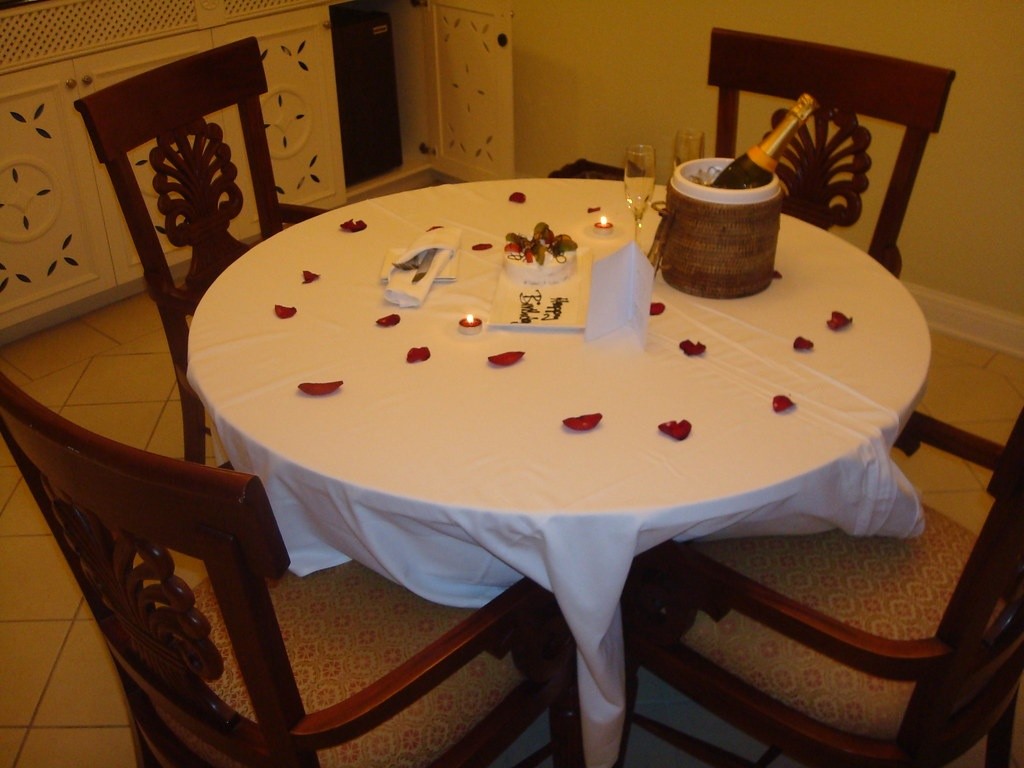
190, 176, 932, 768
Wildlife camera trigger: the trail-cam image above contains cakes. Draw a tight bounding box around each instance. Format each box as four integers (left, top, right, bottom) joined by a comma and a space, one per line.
503, 221, 579, 286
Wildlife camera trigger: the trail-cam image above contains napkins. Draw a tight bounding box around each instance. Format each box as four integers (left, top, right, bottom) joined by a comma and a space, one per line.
379, 225, 461, 308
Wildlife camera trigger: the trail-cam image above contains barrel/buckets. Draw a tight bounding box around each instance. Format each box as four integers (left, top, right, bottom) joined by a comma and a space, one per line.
660, 157, 782, 298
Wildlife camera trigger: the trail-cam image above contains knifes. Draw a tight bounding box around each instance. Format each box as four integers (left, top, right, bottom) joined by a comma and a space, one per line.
412, 248, 437, 283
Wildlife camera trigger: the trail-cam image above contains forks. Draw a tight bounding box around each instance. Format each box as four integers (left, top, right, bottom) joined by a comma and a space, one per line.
391, 255, 419, 271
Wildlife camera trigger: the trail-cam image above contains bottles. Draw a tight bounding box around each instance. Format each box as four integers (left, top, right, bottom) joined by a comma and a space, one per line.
711, 92, 821, 191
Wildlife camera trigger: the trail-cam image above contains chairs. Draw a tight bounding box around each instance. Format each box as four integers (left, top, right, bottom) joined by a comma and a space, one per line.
0, 373, 587, 768
705, 27, 957, 277
71, 35, 329, 465
616, 406, 1024, 768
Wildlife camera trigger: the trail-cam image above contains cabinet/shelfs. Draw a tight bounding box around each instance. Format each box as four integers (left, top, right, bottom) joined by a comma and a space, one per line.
0, 0, 515, 352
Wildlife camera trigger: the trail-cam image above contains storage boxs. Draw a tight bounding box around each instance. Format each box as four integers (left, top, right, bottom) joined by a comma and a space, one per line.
327, 5, 404, 187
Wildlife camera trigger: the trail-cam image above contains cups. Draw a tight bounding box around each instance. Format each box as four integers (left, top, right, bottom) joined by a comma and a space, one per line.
671, 129, 705, 173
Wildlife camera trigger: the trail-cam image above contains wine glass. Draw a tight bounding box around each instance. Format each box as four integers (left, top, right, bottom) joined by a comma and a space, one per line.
623, 145, 655, 247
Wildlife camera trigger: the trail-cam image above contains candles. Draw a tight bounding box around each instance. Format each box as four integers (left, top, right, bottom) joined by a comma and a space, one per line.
592, 215, 614, 235
458, 314, 483, 335
